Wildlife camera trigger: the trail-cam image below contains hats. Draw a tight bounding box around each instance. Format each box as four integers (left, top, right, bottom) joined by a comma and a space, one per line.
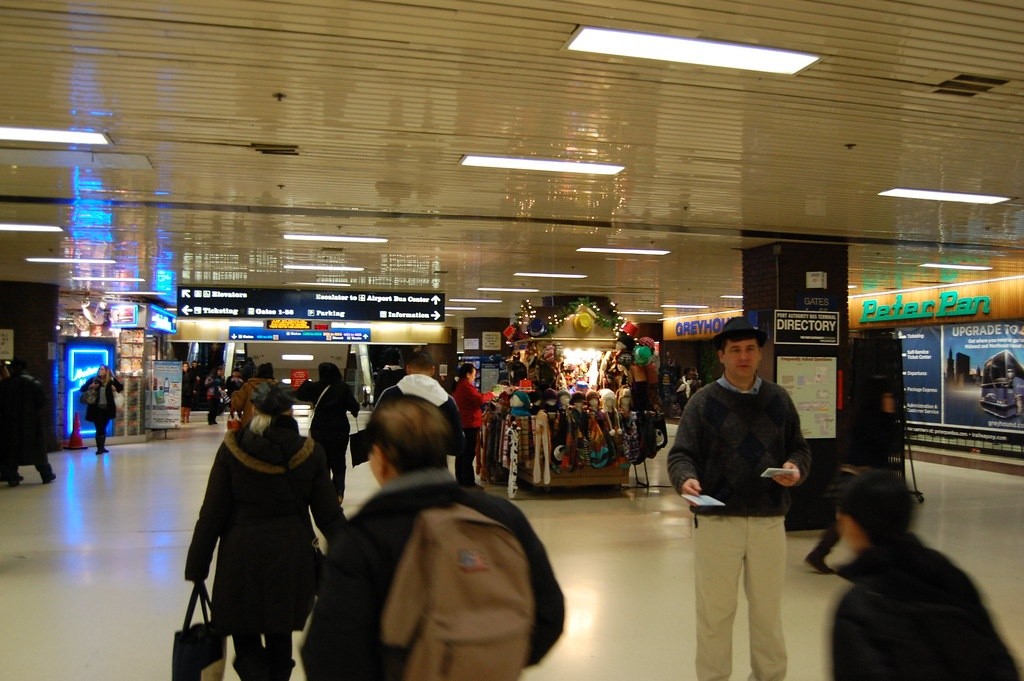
684, 367, 692, 374
618, 354, 632, 366
713, 317, 767, 347
616, 336, 635, 351
639, 336, 654, 348
255, 364, 273, 378
631, 346, 652, 366
528, 319, 547, 337
498, 387, 634, 410
623, 321, 640, 338
251, 383, 295, 414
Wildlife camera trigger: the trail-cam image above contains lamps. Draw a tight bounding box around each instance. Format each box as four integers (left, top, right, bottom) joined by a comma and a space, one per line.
55, 323, 63, 331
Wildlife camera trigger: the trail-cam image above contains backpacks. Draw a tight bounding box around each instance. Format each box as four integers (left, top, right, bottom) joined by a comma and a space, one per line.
383, 503, 536, 681
509, 357, 528, 386
526, 355, 555, 389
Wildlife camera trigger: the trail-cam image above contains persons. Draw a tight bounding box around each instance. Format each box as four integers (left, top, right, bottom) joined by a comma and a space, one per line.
832, 474, 1021, 681
805, 375, 898, 572
367, 351, 465, 470
301, 396, 563, 681
676, 367, 702, 415
0, 357, 56, 487
231, 362, 293, 428
668, 317, 811, 681
181, 361, 243, 425
185, 382, 348, 681
296, 362, 360, 504
510, 388, 631, 415
449, 363, 499, 490
80, 366, 123, 455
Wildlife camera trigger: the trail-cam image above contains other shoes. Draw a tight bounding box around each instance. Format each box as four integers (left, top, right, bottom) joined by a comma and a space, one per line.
805, 552, 833, 573
9, 480, 18, 484
43, 473, 55, 483
96, 447, 109, 456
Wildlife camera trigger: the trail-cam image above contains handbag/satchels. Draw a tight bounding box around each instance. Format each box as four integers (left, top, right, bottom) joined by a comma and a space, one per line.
315, 549, 324, 588
80, 377, 98, 403
219, 388, 231, 404
503, 324, 518, 340
350, 429, 369, 467
172, 580, 226, 681
308, 410, 314, 430
553, 408, 670, 474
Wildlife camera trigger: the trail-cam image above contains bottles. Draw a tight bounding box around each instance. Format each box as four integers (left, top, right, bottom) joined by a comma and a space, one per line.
159, 385, 163, 390
154, 376, 157, 390
164, 378, 169, 396
